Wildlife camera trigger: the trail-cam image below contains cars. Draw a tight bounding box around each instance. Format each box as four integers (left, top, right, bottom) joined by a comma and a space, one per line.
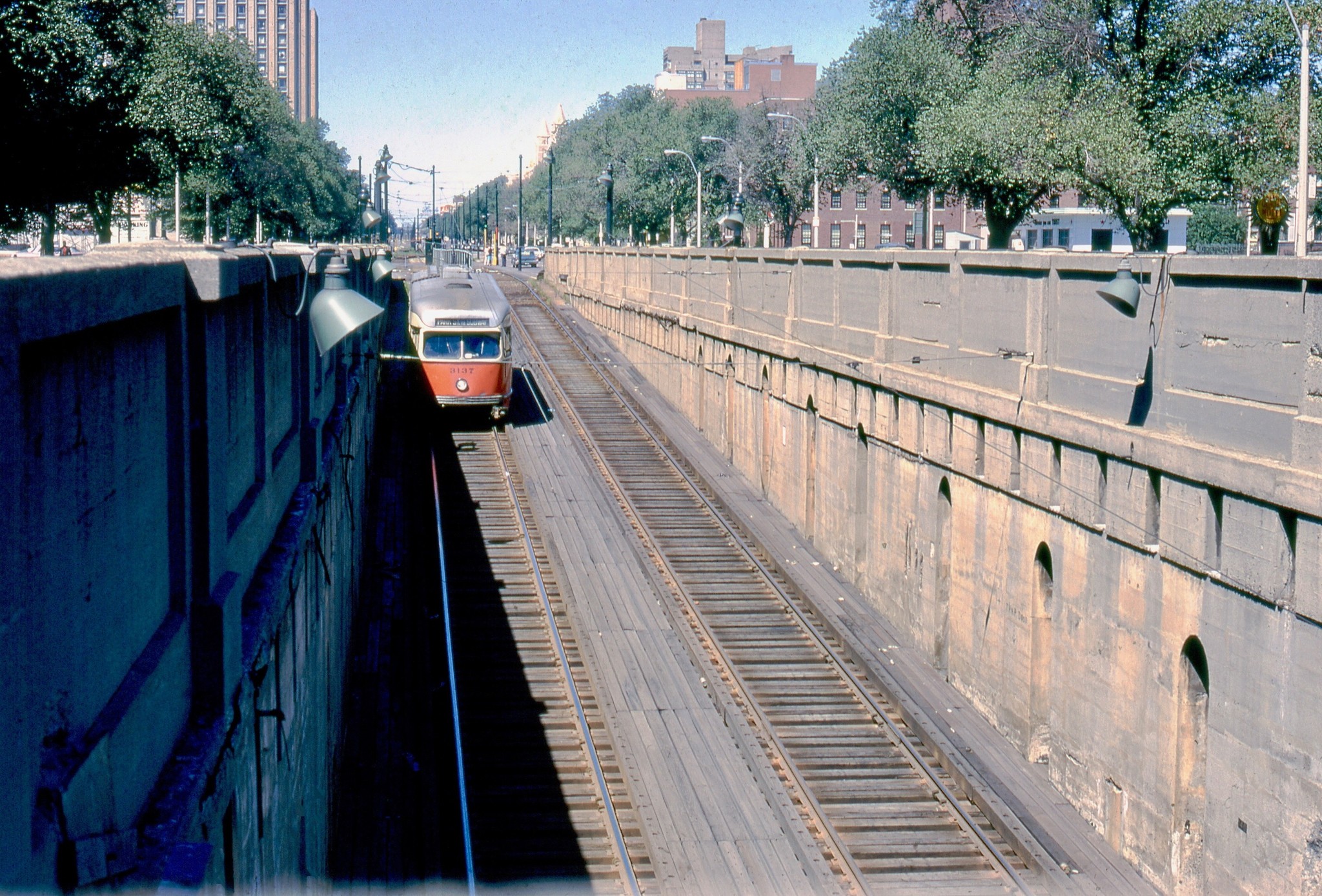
452, 244, 546, 268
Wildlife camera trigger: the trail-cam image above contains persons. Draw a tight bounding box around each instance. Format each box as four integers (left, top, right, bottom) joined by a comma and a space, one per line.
60, 241, 72, 256
484, 244, 492, 266
498, 244, 507, 267
415, 242, 422, 253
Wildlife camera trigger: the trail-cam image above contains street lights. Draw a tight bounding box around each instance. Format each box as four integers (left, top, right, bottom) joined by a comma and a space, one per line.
600, 163, 614, 245
766, 113, 820, 248
379, 144, 393, 225
722, 192, 744, 247
374, 160, 392, 241
663, 149, 702, 247
542, 146, 556, 245
700, 136, 744, 195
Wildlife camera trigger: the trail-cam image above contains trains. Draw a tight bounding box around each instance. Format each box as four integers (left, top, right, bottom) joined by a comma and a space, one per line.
407, 263, 514, 422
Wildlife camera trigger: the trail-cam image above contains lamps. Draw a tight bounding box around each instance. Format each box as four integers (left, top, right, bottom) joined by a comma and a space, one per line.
220, 234, 398, 359
1093, 249, 1187, 319
361, 197, 381, 234
